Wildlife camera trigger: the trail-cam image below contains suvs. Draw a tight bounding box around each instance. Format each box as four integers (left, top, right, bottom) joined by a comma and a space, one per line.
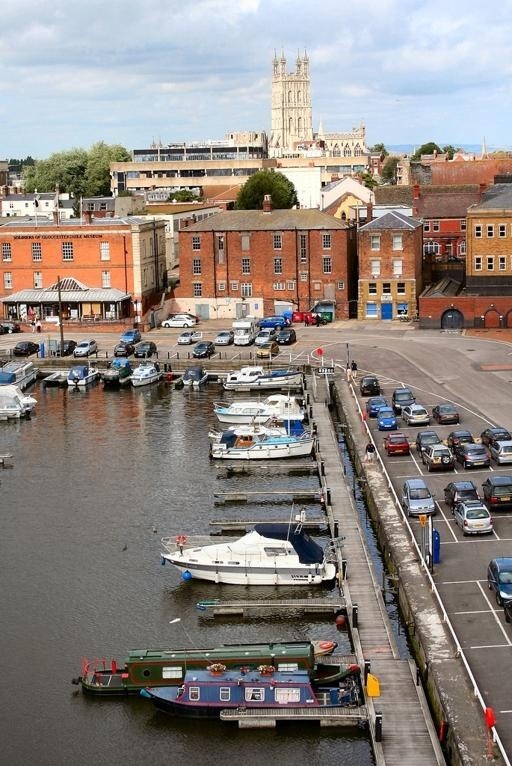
443, 480, 477, 507
120, 330, 142, 343
391, 387, 416, 415
487, 557, 512, 606
421, 445, 455, 472
453, 500, 494, 536
481, 475, 512, 512
359, 376, 380, 396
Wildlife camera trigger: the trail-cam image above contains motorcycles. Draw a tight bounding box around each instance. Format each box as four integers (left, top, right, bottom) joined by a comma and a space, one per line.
311, 316, 328, 325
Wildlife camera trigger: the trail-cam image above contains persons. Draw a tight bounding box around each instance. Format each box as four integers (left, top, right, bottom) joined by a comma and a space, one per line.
315, 315, 320, 327
305, 315, 309, 327
448, 435, 454, 452
365, 440, 375, 463
346, 363, 351, 382
351, 360, 357, 378
27, 309, 41, 333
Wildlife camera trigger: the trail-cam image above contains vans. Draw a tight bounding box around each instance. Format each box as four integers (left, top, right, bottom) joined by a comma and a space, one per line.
401, 478, 436, 518
74, 339, 98, 357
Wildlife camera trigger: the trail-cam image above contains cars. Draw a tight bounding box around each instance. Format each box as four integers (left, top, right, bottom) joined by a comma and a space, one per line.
377, 407, 398, 431
490, 441, 511, 465
50, 339, 78, 356
503, 599, 511, 623
160, 311, 200, 328
365, 397, 389, 417
114, 342, 135, 358
456, 444, 490, 469
2, 321, 21, 333
382, 432, 411, 457
401, 403, 430, 426
431, 403, 459, 424
415, 430, 443, 457
176, 330, 203, 344
214, 313, 296, 357
14, 341, 40, 357
480, 427, 511, 449
447, 430, 473, 454
134, 341, 157, 358
192, 340, 215, 358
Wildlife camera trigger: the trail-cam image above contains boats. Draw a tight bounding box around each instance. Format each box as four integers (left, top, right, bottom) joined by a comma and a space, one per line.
222, 365, 302, 390
70, 635, 363, 700
1, 360, 40, 390
137, 660, 360, 720
157, 503, 340, 588
207, 392, 315, 461
170, 580, 323, 607
43, 358, 164, 386
183, 365, 209, 385
0, 384, 39, 420
309, 638, 339, 660
